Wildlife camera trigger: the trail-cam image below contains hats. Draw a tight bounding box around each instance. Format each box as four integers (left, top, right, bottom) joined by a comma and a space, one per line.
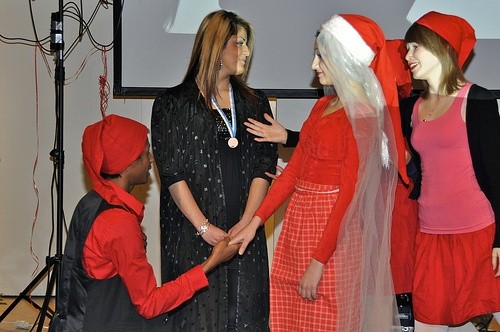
82, 114, 148, 226
415, 11, 477, 72
321, 14, 410, 184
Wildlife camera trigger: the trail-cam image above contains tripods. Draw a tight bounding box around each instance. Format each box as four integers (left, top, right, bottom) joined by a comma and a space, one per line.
0, 0, 66, 332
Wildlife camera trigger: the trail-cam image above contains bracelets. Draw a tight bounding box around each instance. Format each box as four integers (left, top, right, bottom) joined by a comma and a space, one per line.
193, 219, 213, 234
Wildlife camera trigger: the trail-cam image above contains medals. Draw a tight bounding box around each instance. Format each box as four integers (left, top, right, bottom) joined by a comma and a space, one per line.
228, 137, 239, 148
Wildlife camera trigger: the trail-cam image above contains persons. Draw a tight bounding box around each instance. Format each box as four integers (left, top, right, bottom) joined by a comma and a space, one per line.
48, 114, 243, 332
244, 10, 500, 332
228, 13, 411, 332
151, 10, 279, 332
